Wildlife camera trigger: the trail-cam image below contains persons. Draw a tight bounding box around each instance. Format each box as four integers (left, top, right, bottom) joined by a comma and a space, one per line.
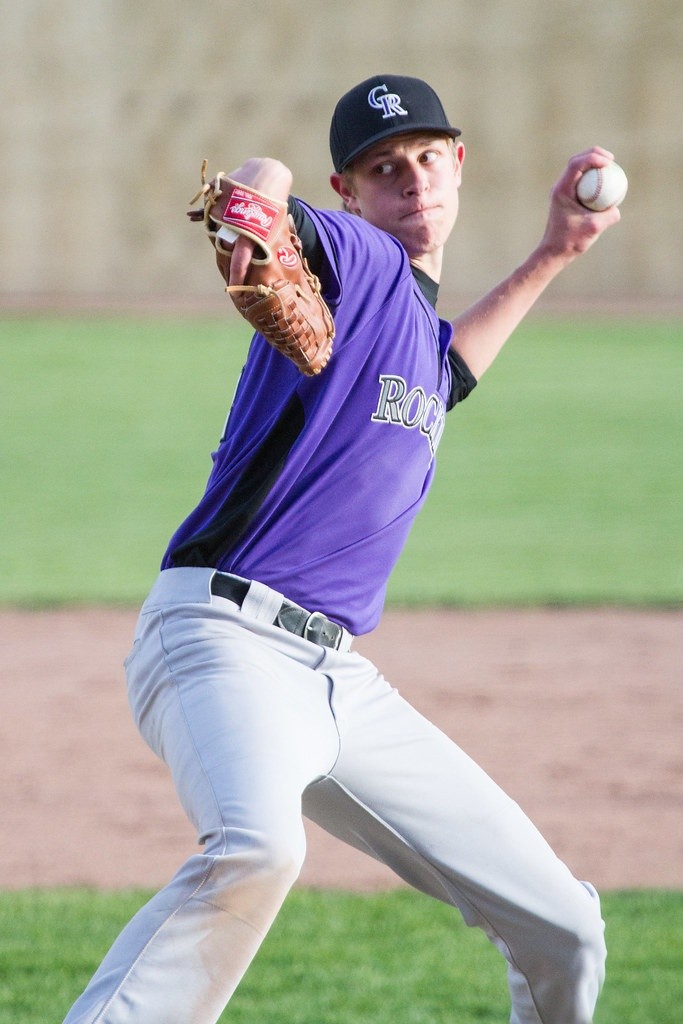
64, 73, 622, 1024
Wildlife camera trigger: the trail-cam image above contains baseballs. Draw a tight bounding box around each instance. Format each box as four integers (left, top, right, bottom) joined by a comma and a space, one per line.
577, 160, 629, 212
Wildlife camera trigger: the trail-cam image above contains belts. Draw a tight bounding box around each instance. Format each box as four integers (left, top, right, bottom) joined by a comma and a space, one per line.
210, 571, 356, 656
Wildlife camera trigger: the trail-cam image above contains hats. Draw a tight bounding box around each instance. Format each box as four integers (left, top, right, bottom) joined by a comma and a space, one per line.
329, 74, 463, 175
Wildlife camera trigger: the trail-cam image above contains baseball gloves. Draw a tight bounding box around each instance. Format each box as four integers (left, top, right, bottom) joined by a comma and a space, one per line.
186, 158, 336, 378
